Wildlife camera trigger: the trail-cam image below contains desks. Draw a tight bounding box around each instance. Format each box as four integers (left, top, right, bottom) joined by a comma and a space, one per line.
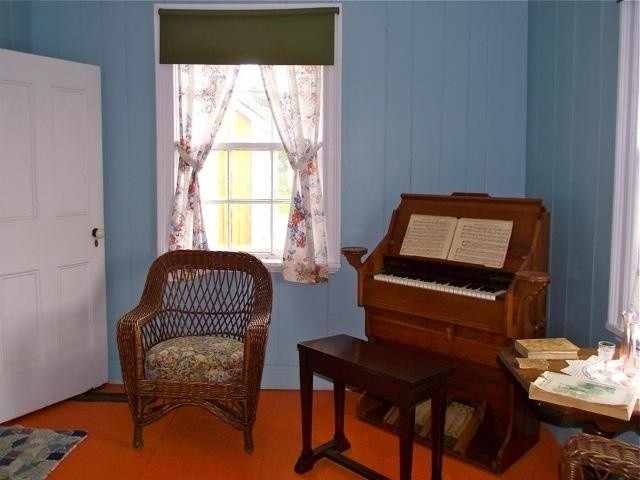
496, 347, 640, 438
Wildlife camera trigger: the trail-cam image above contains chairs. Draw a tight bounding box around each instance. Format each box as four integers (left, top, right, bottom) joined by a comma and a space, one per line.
559, 427, 640, 480
116, 250, 272, 453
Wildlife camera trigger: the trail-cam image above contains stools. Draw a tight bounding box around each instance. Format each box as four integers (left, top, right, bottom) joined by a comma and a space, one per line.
294, 334, 459, 480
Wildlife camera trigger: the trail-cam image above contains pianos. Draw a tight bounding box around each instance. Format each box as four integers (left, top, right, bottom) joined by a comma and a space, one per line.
341, 193, 551, 473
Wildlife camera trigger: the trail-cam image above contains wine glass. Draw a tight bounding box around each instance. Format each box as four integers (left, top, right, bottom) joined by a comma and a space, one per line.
598, 341, 616, 377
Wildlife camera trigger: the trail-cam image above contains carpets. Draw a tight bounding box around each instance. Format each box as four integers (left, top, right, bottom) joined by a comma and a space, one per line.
0, 425, 89, 480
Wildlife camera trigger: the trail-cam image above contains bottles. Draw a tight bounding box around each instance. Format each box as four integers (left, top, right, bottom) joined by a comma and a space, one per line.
621, 310, 640, 381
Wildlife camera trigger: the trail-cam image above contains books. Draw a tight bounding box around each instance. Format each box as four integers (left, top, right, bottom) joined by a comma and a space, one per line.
527, 370, 639, 421
514, 342, 578, 360
515, 357, 550, 370
516, 336, 580, 352
399, 212, 514, 269
